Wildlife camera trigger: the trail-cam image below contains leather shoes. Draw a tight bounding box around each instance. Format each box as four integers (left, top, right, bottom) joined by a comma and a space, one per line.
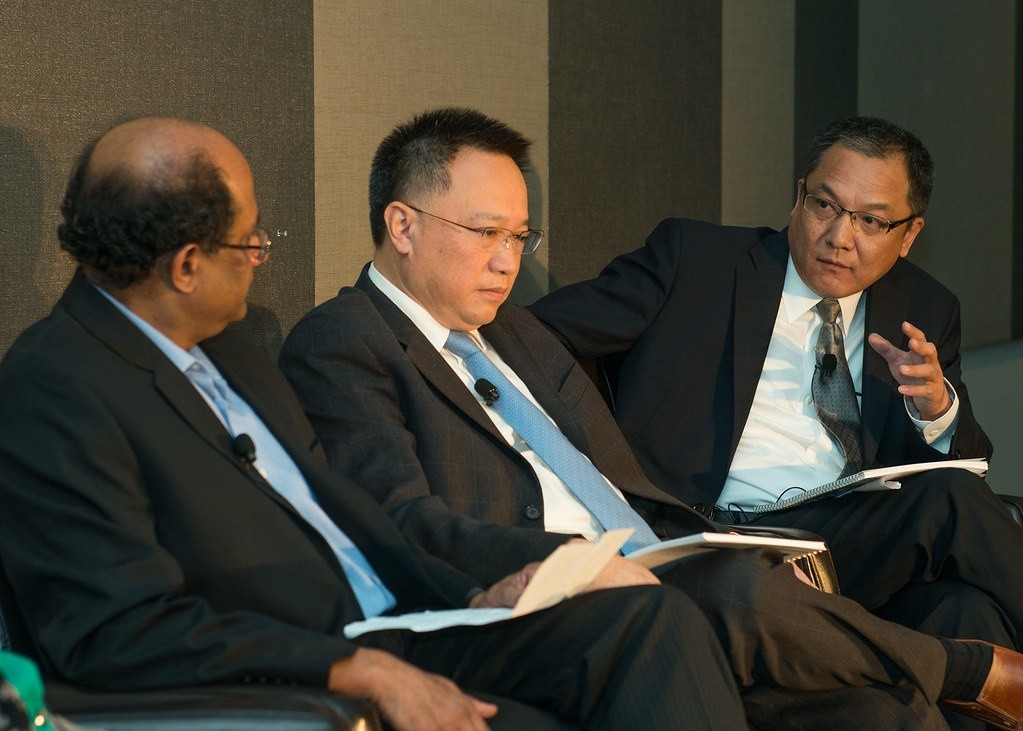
939, 637, 1023, 731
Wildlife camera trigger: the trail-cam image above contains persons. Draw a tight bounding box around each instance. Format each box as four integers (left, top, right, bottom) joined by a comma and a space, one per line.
0, 114, 754, 731
282, 104, 955, 731
525, 109, 1023, 653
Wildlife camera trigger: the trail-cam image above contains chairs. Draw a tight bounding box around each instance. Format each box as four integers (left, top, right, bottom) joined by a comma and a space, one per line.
0, 636, 385, 730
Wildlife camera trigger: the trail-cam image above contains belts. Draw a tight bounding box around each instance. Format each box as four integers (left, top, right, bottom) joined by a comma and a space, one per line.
710, 508, 765, 525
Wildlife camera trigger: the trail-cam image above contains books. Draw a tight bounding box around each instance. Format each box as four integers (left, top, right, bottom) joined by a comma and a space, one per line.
751, 455, 989, 518
621, 530, 828, 577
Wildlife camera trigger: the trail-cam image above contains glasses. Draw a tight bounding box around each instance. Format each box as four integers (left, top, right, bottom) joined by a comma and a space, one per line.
403, 203, 544, 255
801, 175, 920, 237
212, 225, 271, 261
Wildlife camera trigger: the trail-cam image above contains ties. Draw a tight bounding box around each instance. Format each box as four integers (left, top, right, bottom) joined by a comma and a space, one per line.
445, 329, 661, 556
810, 298, 863, 499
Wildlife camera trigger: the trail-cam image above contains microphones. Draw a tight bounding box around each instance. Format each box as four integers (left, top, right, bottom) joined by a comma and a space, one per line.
233, 432, 257, 466
474, 378, 501, 407
823, 353, 837, 378
